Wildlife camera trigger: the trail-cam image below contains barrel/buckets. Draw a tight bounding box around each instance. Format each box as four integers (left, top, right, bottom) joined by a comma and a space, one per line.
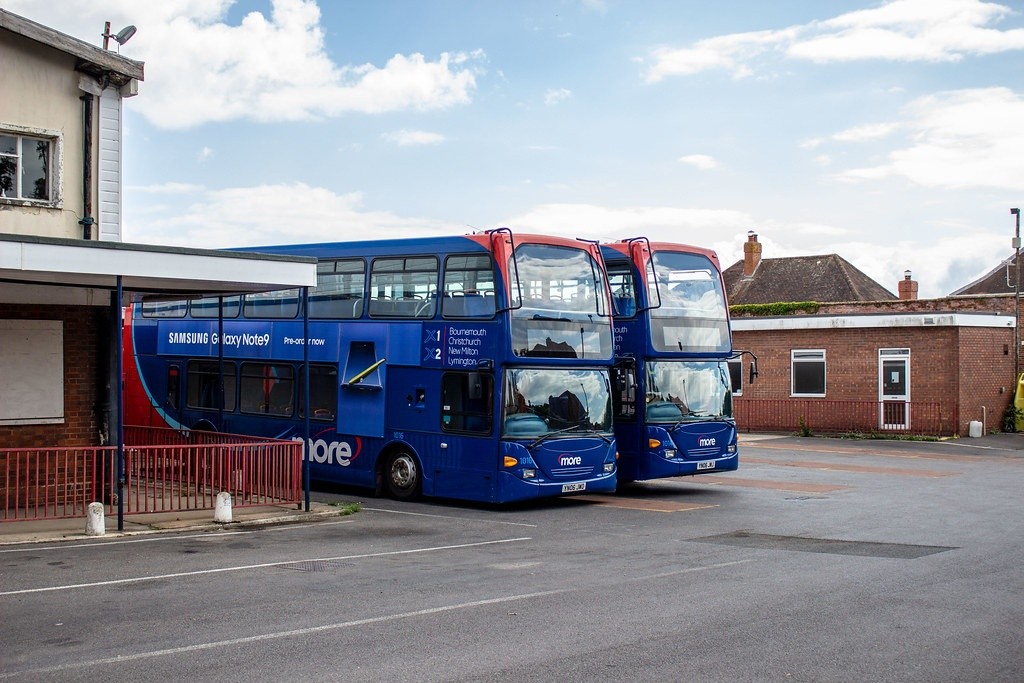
969, 419, 983, 438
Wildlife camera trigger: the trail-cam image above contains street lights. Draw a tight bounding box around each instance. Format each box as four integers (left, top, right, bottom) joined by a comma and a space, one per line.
1010, 207, 1020, 393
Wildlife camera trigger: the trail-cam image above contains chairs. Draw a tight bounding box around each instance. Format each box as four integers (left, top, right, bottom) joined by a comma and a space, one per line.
514, 293, 594, 312
452, 291, 464, 315
611, 293, 621, 317
281, 291, 298, 316
393, 297, 412, 315
464, 289, 490, 317
177, 301, 186, 316
253, 294, 272, 317
377, 295, 395, 315
314, 409, 332, 419
431, 290, 452, 316
347, 295, 362, 318
227, 298, 238, 316
202, 298, 218, 316
155, 302, 166, 317
485, 291, 496, 315
620, 294, 636, 319
168, 301, 177, 316
309, 294, 328, 317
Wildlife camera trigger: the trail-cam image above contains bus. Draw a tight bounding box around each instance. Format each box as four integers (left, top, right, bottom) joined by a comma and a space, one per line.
600, 237, 760, 493
120, 229, 618, 507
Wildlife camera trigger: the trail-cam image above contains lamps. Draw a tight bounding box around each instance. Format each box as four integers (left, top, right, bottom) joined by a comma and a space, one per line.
101, 24, 137, 45
